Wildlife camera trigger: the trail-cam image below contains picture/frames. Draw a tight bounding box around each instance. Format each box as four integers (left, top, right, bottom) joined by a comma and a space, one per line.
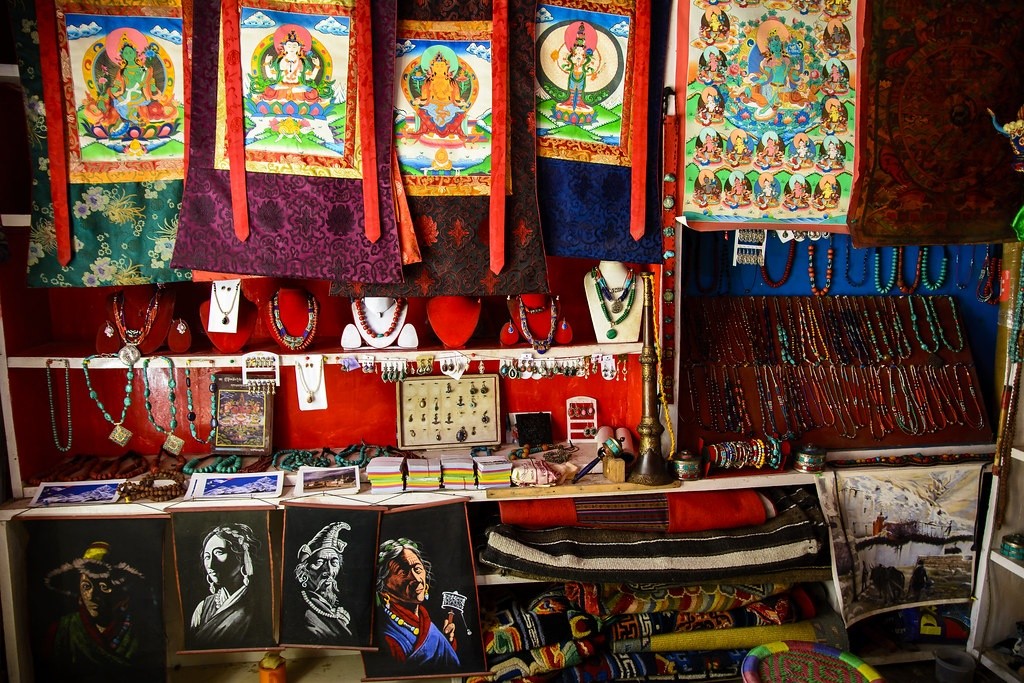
214, 372, 273, 456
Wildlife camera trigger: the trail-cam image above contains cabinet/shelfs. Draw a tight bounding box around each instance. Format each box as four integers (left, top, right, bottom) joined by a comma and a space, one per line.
0, 461, 994, 682
967, 360, 1024, 683
0, 1, 681, 499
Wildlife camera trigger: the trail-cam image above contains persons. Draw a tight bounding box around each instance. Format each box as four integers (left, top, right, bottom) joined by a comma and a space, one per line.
861, 561, 869, 591
910, 560, 928, 601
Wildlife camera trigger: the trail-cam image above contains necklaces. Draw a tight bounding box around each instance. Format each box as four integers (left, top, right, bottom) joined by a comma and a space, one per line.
355, 297, 403, 339
112, 286, 162, 365
756, 229, 1002, 305
182, 358, 216, 444
46, 359, 72, 452
269, 292, 318, 350
517, 296, 559, 355
212, 281, 241, 326
468, 440, 580, 467
82, 352, 134, 448
679, 294, 983, 472
590, 266, 637, 339
29, 441, 409, 504
143, 356, 186, 456
